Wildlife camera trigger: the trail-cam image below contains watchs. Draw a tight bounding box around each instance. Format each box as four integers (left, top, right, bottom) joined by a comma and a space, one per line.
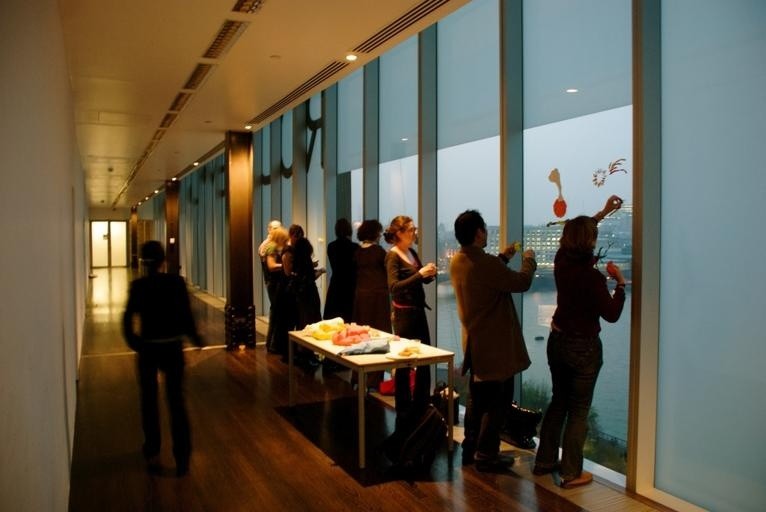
616, 284, 625, 287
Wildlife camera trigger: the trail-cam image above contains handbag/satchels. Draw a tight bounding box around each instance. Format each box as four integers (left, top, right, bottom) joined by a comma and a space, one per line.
434, 384, 460, 425
502, 402, 542, 449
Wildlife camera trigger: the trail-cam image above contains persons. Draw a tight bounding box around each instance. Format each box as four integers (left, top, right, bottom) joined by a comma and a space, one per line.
290, 239, 327, 358
319, 216, 361, 375
446, 208, 537, 473
533, 195, 629, 490
266, 220, 282, 236
263, 228, 290, 354
352, 218, 388, 393
122, 239, 208, 477
382, 216, 438, 433
281, 224, 319, 365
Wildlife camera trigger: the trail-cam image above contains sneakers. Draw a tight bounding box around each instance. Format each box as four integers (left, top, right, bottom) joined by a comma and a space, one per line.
462, 450, 475, 465
560, 472, 592, 488
533, 460, 561, 475
474, 452, 514, 472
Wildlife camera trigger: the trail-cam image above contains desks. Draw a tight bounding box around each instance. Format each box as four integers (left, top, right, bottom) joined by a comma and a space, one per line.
287, 321, 455, 470
